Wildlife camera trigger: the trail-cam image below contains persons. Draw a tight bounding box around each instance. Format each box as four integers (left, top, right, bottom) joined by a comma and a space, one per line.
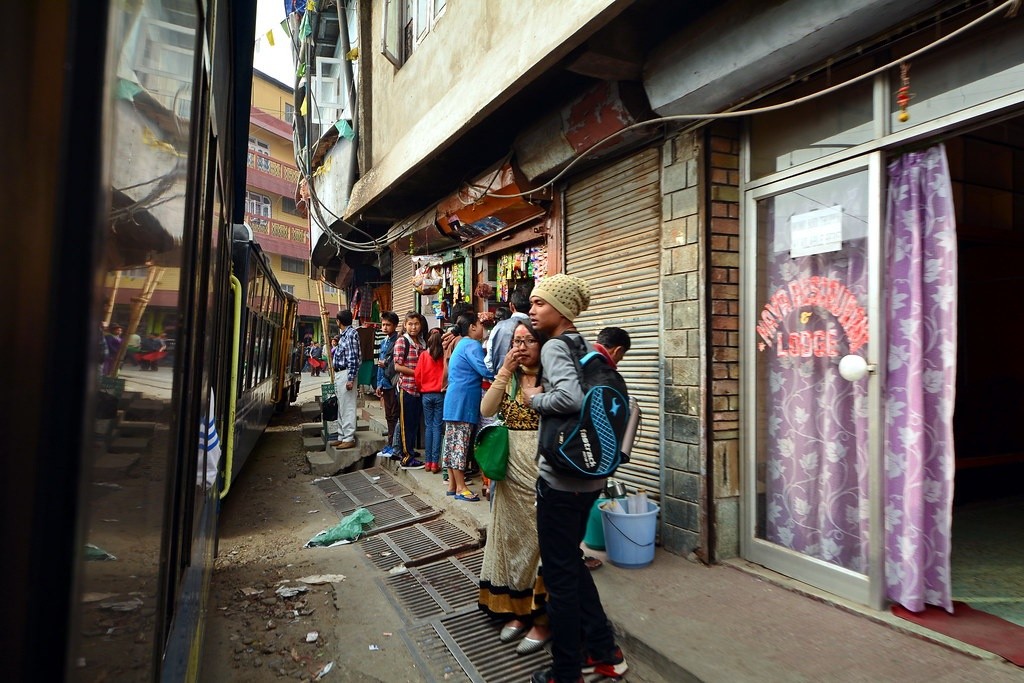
440, 289, 532, 501
330, 310, 362, 449
523, 274, 628, 683
478, 320, 551, 654
376, 311, 400, 458
391, 311, 428, 470
576, 327, 630, 571
414, 327, 449, 473
296, 335, 340, 377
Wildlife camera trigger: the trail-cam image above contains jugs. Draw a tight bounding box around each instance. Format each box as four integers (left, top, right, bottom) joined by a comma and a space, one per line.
619, 394, 642, 463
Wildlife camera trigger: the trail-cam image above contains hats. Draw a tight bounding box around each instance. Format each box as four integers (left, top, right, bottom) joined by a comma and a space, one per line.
529, 273, 590, 322
331, 335, 340, 342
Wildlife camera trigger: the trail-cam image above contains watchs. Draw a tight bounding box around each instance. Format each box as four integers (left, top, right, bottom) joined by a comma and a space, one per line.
528, 394, 534, 406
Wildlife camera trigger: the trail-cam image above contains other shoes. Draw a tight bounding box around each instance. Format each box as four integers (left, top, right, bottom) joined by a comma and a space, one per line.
500, 626, 526, 643
443, 474, 473, 485
425, 462, 431, 472
330, 440, 342, 446
431, 462, 441, 474
337, 442, 356, 449
515, 636, 544, 654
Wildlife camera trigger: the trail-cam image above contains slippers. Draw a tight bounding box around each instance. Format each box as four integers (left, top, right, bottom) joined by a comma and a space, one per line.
581, 555, 602, 570
454, 490, 480, 501
446, 491, 455, 496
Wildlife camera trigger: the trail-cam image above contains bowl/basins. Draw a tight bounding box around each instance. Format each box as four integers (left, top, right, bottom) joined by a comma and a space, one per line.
604, 482, 626, 497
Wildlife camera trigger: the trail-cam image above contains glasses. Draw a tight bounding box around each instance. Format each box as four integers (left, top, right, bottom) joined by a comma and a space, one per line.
510, 338, 535, 347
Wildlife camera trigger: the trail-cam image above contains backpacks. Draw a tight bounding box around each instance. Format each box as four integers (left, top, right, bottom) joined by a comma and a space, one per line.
534, 335, 643, 480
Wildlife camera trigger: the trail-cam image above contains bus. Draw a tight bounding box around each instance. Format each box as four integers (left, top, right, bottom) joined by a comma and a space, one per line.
0, 0, 305, 683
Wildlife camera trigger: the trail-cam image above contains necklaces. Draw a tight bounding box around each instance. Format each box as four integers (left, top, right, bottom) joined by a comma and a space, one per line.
524, 374, 535, 387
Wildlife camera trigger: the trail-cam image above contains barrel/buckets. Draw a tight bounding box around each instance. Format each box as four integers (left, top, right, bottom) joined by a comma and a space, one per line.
598, 498, 660, 569
582, 495, 628, 550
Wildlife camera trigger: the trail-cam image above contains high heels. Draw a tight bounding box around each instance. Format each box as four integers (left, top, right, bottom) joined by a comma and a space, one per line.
486, 489, 490, 501
481, 484, 487, 497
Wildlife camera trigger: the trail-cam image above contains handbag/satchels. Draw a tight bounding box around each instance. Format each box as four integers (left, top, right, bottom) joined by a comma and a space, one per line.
384, 335, 410, 381
322, 391, 338, 421
471, 372, 517, 481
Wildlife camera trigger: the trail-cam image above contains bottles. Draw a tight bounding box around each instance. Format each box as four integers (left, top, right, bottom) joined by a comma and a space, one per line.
600, 488, 648, 514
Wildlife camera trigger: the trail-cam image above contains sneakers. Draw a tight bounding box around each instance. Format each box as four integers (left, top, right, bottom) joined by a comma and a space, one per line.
580, 644, 629, 678
384, 448, 401, 457
530, 671, 584, 683
377, 445, 392, 456
409, 452, 423, 457
400, 457, 425, 470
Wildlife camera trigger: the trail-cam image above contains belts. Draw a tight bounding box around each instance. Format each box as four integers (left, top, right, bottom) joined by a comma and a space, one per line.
334, 366, 346, 372
